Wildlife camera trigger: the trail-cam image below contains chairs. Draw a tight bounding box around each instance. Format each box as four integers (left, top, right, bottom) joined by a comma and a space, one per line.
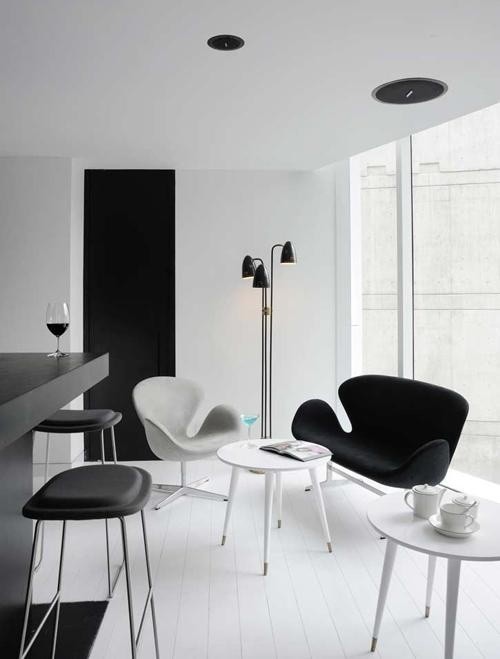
130, 374, 248, 511
288, 373, 470, 541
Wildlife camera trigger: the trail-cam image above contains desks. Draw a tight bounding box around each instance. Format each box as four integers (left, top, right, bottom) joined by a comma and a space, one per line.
216, 438, 335, 577
365, 484, 500, 659
0, 350, 111, 659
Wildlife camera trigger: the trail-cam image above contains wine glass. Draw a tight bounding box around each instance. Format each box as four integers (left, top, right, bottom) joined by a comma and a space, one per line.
239, 413, 260, 448
46, 302, 70, 357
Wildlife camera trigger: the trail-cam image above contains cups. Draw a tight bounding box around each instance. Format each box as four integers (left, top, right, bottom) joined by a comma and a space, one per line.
440, 503, 473, 529
452, 495, 480, 518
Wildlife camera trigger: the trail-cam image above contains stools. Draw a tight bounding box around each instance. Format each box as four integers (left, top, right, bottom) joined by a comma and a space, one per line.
30, 408, 128, 600
17, 462, 161, 659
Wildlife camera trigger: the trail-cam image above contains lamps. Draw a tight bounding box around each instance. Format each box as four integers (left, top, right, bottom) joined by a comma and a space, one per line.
251, 263, 270, 439
263, 240, 298, 438
240, 253, 264, 439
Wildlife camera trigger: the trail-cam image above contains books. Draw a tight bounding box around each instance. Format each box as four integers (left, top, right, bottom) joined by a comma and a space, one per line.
261, 441, 336, 462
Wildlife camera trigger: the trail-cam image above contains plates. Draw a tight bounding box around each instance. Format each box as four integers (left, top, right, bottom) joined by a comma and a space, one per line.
428, 513, 481, 537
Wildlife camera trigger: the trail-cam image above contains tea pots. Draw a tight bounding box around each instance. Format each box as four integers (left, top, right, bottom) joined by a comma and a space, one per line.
404, 483, 447, 518
